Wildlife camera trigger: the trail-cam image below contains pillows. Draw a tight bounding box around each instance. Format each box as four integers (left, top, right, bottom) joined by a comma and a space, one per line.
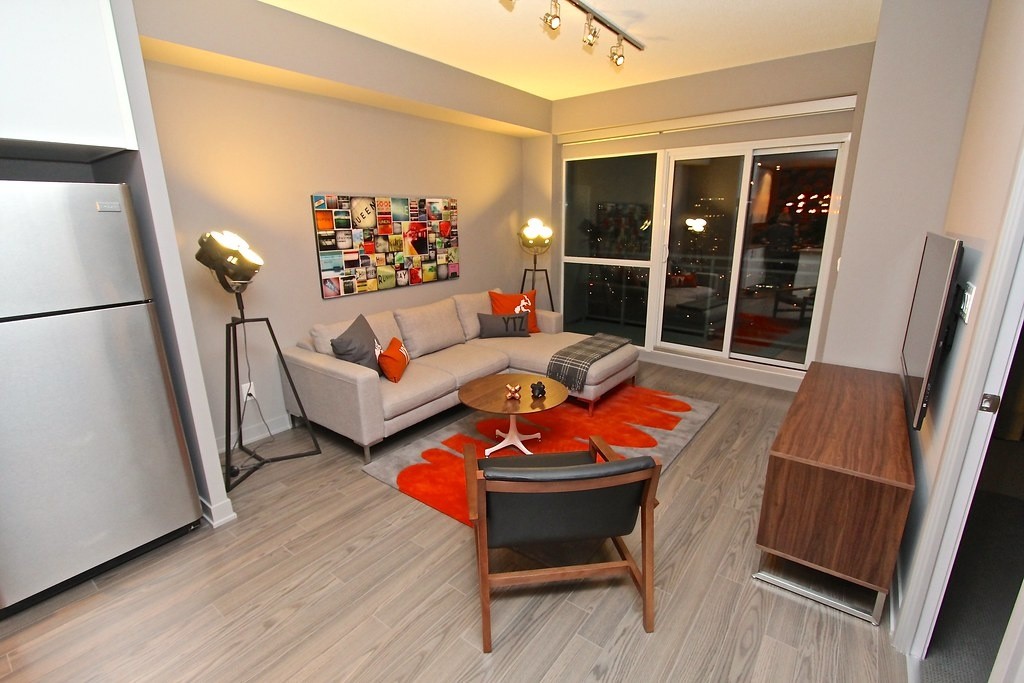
330, 314, 383, 377
477, 311, 531, 340
488, 289, 541, 333
378, 337, 410, 383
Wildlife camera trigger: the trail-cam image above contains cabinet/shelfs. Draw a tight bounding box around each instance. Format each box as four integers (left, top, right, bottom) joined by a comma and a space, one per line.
750, 362, 915, 625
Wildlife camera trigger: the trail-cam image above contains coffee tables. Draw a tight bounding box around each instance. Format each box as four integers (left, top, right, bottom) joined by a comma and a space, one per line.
458, 374, 568, 459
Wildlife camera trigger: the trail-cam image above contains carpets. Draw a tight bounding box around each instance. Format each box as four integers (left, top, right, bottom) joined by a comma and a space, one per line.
360, 383, 720, 569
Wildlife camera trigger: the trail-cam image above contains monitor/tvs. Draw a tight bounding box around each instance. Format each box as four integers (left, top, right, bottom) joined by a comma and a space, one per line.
901, 231, 966, 434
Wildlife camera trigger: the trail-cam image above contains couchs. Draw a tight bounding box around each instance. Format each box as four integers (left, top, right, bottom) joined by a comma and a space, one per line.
277, 288, 639, 466
604, 266, 729, 334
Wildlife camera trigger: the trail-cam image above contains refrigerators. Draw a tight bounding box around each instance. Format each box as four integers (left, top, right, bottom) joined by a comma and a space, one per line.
0, 180, 203, 618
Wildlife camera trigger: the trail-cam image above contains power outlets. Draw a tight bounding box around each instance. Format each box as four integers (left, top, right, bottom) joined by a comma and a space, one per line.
242, 382, 256, 402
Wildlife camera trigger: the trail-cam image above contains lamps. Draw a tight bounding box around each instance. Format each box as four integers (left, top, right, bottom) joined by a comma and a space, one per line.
609, 34, 625, 67
543, 0, 561, 30
582, 13, 600, 46
196, 231, 264, 319
516, 217, 553, 270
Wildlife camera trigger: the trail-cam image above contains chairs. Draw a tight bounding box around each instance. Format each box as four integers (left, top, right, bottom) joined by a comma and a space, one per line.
773, 286, 815, 324
464, 436, 662, 653
